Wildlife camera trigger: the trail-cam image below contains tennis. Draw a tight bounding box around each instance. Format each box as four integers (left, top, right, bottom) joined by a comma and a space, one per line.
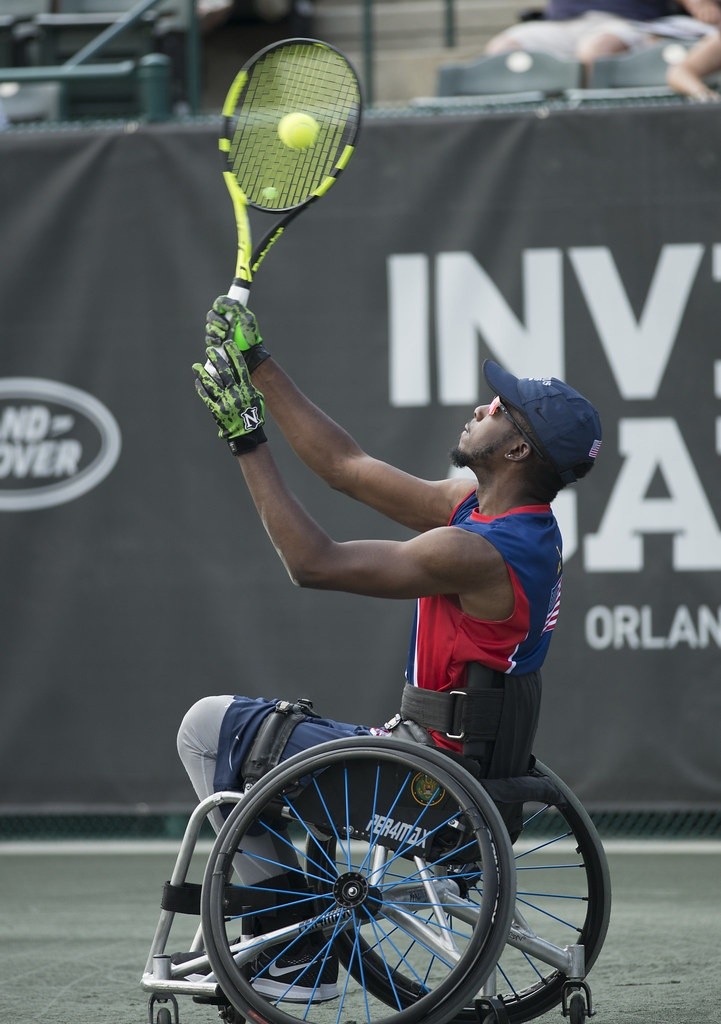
277, 111, 321, 152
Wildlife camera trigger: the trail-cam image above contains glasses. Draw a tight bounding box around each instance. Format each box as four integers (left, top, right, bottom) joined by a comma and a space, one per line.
488, 396, 546, 463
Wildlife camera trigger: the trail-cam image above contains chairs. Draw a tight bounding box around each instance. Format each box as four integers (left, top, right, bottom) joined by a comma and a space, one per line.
590, 40, 721, 92
435, 47, 586, 102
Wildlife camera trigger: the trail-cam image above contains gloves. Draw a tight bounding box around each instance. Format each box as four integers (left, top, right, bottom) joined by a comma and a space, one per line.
205, 296, 272, 375
192, 339, 267, 457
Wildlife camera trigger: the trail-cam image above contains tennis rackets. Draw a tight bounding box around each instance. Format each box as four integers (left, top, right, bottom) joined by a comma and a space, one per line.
198, 34, 365, 394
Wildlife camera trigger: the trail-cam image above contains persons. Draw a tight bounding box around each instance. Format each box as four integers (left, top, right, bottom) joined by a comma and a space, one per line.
550, 0, 721, 101
176, 296, 602, 1001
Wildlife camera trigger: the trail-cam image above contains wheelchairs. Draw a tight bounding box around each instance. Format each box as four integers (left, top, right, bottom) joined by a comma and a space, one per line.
136, 660, 613, 1024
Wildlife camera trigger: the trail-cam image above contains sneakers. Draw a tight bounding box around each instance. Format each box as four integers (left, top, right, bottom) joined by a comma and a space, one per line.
170, 934, 338, 1006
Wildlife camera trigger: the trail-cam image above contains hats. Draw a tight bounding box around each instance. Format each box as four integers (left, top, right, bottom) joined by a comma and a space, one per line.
482, 358, 602, 483
